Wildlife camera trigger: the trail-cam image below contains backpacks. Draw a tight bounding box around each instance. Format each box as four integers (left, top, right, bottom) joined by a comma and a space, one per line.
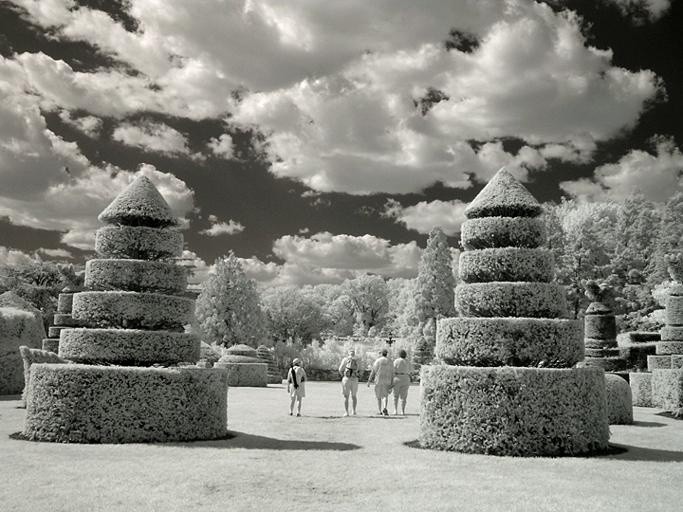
344, 357, 357, 377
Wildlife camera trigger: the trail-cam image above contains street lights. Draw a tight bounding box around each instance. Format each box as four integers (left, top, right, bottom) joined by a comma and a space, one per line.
388, 331, 392, 347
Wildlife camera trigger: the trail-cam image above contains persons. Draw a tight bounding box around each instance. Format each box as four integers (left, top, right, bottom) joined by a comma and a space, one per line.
339, 348, 364, 416
286, 357, 307, 417
367, 349, 410, 417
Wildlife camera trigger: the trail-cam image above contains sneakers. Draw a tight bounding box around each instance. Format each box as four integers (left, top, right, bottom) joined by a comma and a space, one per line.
343, 412, 357, 416
290, 413, 301, 417
376, 408, 405, 415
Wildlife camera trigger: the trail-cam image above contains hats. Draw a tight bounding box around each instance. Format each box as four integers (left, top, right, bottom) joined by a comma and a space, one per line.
293, 358, 301, 364
378, 348, 388, 354
